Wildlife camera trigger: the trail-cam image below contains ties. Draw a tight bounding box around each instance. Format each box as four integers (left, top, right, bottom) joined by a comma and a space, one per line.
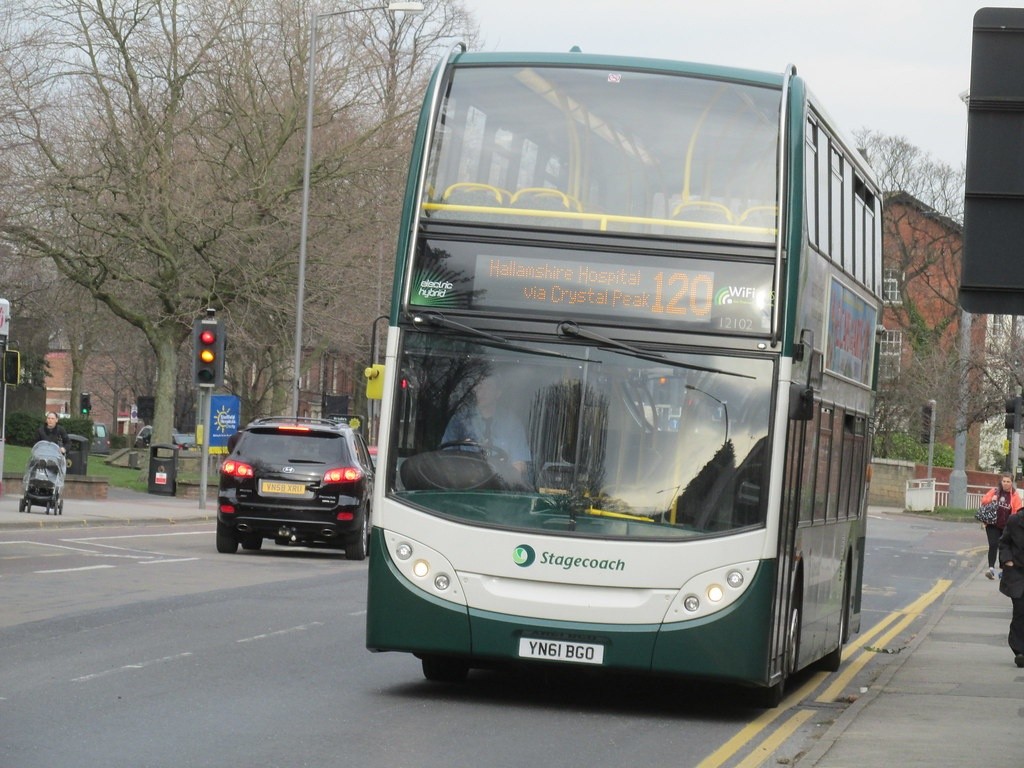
483, 416, 492, 455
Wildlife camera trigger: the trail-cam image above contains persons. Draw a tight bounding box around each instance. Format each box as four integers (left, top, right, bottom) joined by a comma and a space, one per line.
997, 506, 1024, 667
31, 411, 71, 459
440, 372, 533, 476
980, 472, 1022, 581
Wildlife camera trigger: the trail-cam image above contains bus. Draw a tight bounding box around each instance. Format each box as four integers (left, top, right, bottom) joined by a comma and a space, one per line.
363, 43, 884, 694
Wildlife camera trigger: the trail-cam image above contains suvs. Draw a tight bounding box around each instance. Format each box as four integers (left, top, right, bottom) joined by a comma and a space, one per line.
534, 462, 576, 489
217, 416, 378, 559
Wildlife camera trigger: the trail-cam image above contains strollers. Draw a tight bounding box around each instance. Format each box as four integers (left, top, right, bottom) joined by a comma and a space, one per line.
19, 441, 66, 516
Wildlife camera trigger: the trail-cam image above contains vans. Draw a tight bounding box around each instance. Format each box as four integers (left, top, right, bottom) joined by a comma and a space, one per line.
89, 423, 110, 454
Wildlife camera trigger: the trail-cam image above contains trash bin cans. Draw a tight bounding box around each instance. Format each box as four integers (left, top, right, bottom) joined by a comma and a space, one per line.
147, 441, 179, 494
63, 432, 90, 476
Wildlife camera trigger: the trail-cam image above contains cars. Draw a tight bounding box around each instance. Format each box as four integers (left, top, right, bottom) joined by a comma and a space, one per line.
135, 425, 196, 450
367, 445, 378, 469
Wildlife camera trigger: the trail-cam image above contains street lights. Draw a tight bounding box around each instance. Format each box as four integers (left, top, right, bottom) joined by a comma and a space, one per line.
685, 384, 729, 445
291, 2, 428, 420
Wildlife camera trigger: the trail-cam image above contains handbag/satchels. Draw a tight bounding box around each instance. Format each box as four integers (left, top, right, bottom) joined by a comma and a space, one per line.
975, 487, 998, 526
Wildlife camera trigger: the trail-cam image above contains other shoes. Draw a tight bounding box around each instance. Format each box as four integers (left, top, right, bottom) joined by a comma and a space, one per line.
998, 572, 1002, 578
1015, 654, 1024, 668
985, 568, 994, 579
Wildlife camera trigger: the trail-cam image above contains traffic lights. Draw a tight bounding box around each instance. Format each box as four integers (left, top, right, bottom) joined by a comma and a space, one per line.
194, 319, 224, 389
1005, 397, 1022, 433
81, 393, 91, 414
